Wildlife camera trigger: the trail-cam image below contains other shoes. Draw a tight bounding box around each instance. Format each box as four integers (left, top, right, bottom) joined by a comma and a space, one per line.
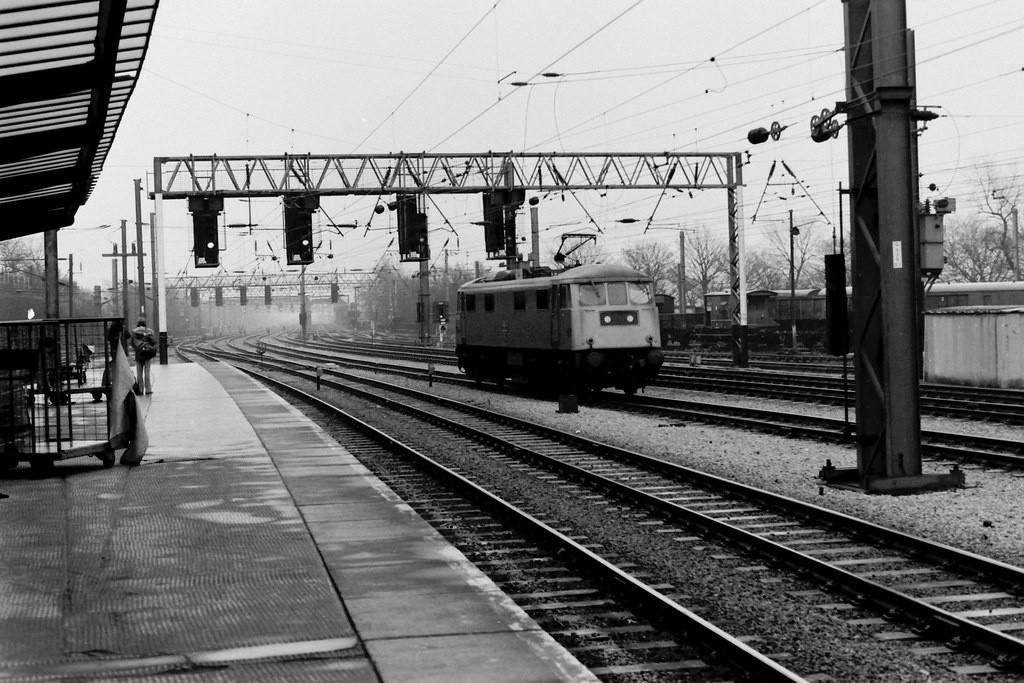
145, 392, 153, 395
135, 392, 144, 395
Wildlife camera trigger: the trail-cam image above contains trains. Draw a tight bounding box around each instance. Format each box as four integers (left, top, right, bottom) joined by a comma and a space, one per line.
653, 281, 1024, 351
455, 260, 665, 396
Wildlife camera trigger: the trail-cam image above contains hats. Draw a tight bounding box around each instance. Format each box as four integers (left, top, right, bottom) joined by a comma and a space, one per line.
137, 317, 145, 323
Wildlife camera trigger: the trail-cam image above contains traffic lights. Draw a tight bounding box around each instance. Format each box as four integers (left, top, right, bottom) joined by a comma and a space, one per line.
238, 286, 249, 307
329, 285, 340, 305
394, 194, 430, 260
190, 288, 198, 307
188, 198, 223, 267
216, 288, 223, 308
262, 285, 273, 305
482, 190, 527, 255
283, 196, 320, 267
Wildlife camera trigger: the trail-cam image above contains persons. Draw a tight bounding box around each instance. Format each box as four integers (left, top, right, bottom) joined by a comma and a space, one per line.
109, 317, 132, 359
130, 316, 156, 395
